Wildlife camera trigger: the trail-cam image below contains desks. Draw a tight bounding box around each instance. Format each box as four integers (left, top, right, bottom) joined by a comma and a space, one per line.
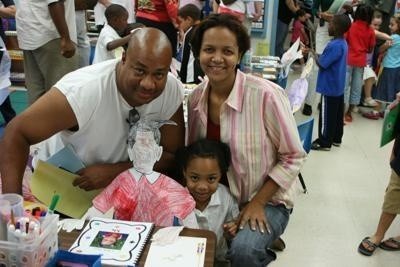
0, 213, 217, 267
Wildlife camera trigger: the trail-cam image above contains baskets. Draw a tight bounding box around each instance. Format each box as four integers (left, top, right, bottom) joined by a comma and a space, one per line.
0, 214, 60, 267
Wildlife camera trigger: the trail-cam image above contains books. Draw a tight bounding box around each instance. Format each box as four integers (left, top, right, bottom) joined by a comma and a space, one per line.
68, 217, 155, 266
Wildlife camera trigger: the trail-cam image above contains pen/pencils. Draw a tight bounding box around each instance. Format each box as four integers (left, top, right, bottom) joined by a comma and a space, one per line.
6, 192, 60, 242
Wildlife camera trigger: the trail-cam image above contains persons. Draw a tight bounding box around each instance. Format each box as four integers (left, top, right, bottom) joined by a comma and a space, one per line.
172, 139, 243, 267
358, 94, 399, 255
98, 229, 128, 247
0, 27, 188, 218
1, 0, 400, 153
184, 12, 309, 266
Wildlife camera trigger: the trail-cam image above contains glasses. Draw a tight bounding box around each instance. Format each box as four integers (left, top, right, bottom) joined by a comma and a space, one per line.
126, 105, 140, 144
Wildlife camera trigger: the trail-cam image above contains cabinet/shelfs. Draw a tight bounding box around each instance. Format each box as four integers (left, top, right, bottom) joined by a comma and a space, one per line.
85, 9, 101, 47
5, 29, 26, 84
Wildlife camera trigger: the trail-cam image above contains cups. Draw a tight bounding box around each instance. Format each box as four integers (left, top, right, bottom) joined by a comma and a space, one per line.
6, 214, 40, 244
0, 193, 24, 240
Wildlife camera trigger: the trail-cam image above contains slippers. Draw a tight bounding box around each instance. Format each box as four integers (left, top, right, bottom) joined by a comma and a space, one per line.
358, 237, 377, 255
380, 237, 400, 250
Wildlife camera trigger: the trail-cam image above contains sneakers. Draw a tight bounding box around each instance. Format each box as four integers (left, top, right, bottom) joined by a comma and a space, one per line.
362, 111, 378, 119
311, 139, 331, 150
332, 138, 341, 146
380, 113, 384, 117
363, 97, 378, 106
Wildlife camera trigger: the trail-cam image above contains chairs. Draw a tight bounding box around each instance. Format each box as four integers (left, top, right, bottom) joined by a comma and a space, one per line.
277, 69, 288, 88
294, 115, 316, 194
